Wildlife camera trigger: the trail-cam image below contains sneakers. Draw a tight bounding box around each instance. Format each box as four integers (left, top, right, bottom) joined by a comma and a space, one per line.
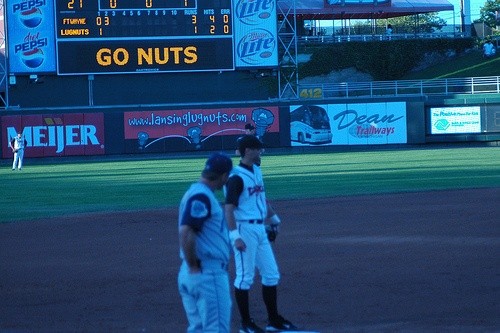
266, 314, 298, 332
239, 319, 265, 333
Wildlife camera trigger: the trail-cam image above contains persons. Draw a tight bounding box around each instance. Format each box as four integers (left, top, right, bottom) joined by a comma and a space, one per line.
235, 123, 264, 167
482, 40, 496, 57
222, 135, 299, 333
176, 151, 233, 333
9, 131, 27, 170
385, 24, 393, 41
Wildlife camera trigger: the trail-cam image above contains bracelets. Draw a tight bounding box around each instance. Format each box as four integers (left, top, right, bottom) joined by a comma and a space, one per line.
270, 214, 281, 225
230, 229, 241, 240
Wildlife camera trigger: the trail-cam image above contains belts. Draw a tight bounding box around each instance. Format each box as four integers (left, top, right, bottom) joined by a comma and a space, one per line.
243, 220, 263, 224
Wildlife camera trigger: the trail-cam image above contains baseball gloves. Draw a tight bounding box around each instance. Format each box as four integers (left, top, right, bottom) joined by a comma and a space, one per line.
266, 224, 279, 242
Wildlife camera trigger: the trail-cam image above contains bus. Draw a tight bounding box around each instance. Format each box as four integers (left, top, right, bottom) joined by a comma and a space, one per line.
290, 105, 332, 144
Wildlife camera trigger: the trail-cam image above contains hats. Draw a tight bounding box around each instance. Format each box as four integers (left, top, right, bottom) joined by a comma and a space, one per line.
236, 135, 267, 148
245, 124, 256, 130
206, 154, 233, 175
